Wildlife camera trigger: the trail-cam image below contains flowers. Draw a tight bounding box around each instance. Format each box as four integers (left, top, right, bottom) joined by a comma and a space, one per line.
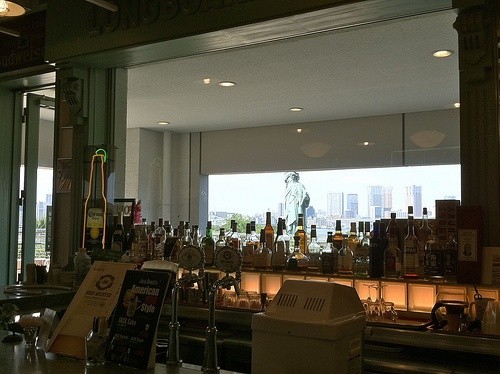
19, 315, 45, 341
0, 303, 19, 327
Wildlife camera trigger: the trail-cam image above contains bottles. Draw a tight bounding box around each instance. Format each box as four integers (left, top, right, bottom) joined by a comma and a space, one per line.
444, 234, 458, 282
111, 211, 385, 278
418, 208, 433, 277
428, 219, 443, 282
74, 249, 91, 287
63, 257, 74, 272
386, 213, 401, 249
383, 226, 402, 279
85, 317, 104, 365
403, 206, 419, 276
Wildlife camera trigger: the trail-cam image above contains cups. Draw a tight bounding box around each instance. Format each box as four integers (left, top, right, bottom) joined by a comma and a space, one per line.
360, 302, 396, 322
223, 290, 276, 310
447, 305, 460, 331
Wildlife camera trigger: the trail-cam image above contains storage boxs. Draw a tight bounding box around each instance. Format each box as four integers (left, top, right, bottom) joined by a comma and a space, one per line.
456, 205, 500, 286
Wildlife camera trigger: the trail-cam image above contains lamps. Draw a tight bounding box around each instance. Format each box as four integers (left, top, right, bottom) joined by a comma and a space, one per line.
0, 0, 26, 17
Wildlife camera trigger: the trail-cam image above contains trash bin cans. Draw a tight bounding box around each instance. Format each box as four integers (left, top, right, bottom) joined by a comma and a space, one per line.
250, 281, 366, 374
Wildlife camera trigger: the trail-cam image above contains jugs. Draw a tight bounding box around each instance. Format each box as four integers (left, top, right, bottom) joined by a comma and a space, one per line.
469, 298, 496, 323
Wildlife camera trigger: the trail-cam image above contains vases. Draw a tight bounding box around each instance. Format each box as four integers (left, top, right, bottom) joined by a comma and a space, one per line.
23, 327, 38, 343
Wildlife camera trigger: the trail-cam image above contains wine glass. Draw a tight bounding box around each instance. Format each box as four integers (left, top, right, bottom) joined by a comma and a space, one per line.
363, 284, 387, 303
